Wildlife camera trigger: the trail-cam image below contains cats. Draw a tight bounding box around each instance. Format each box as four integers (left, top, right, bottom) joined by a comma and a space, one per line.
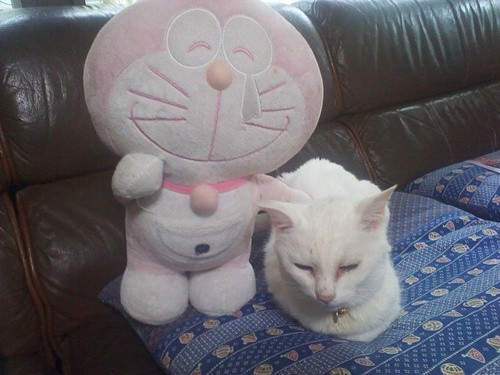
257, 156, 402, 343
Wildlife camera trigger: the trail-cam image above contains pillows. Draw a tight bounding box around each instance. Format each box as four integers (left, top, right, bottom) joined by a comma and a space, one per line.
99, 190, 498, 375
405, 150, 500, 221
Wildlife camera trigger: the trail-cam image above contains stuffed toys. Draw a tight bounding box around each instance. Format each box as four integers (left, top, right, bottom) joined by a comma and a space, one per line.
83, 0, 326, 327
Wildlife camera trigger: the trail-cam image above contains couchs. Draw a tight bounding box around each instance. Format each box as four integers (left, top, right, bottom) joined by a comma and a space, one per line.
0, 0, 500, 375
